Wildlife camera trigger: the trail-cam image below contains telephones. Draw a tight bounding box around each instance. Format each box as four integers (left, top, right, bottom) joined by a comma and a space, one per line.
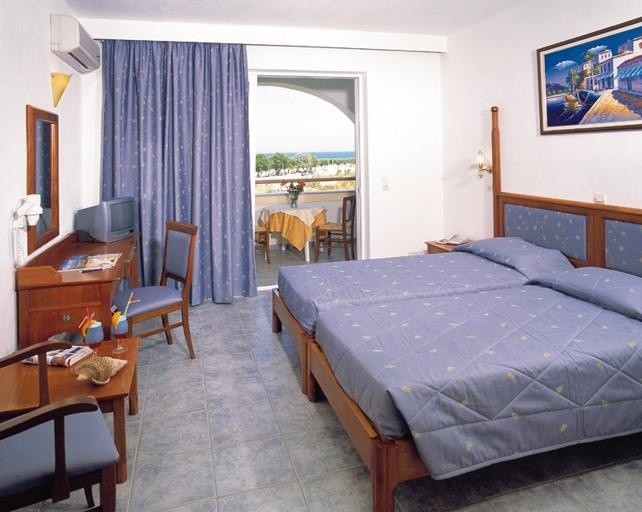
436, 234, 468, 245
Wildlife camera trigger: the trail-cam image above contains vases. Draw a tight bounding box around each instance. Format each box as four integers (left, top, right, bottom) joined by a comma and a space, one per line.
287, 192, 298, 208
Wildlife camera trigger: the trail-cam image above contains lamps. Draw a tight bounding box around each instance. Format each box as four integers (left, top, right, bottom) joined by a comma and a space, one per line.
469, 148, 493, 181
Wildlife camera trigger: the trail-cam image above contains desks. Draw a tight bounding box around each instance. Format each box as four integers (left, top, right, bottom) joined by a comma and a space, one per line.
261, 204, 325, 263
0, 336, 141, 482
15, 232, 139, 352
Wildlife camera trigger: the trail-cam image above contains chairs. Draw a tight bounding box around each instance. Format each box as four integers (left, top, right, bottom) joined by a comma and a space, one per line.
0, 340, 121, 512
112, 221, 198, 360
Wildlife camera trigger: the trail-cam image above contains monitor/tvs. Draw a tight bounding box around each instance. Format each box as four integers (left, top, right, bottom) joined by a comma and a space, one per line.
74, 197, 137, 242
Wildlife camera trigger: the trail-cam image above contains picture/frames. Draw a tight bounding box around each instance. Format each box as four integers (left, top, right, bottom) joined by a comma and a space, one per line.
535, 16, 642, 136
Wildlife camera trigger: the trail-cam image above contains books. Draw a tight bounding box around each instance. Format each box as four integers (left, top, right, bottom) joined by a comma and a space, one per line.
56, 252, 123, 270
21, 344, 95, 367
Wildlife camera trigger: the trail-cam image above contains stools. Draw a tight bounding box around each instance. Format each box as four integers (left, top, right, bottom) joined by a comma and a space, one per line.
254, 226, 270, 264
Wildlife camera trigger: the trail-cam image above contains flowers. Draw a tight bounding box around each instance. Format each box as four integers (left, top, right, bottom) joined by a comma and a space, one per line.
279, 179, 306, 193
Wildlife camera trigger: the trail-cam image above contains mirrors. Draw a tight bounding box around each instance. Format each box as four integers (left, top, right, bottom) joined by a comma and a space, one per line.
24, 104, 60, 255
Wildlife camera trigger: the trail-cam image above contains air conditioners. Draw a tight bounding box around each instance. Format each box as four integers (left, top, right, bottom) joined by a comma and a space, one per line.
48, 12, 100, 74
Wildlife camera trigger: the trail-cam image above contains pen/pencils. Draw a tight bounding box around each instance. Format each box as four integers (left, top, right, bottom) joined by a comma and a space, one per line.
82, 268, 102, 273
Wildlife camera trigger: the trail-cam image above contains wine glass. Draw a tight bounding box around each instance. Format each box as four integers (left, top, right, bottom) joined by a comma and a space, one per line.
86, 322, 104, 358
111, 316, 129, 354
